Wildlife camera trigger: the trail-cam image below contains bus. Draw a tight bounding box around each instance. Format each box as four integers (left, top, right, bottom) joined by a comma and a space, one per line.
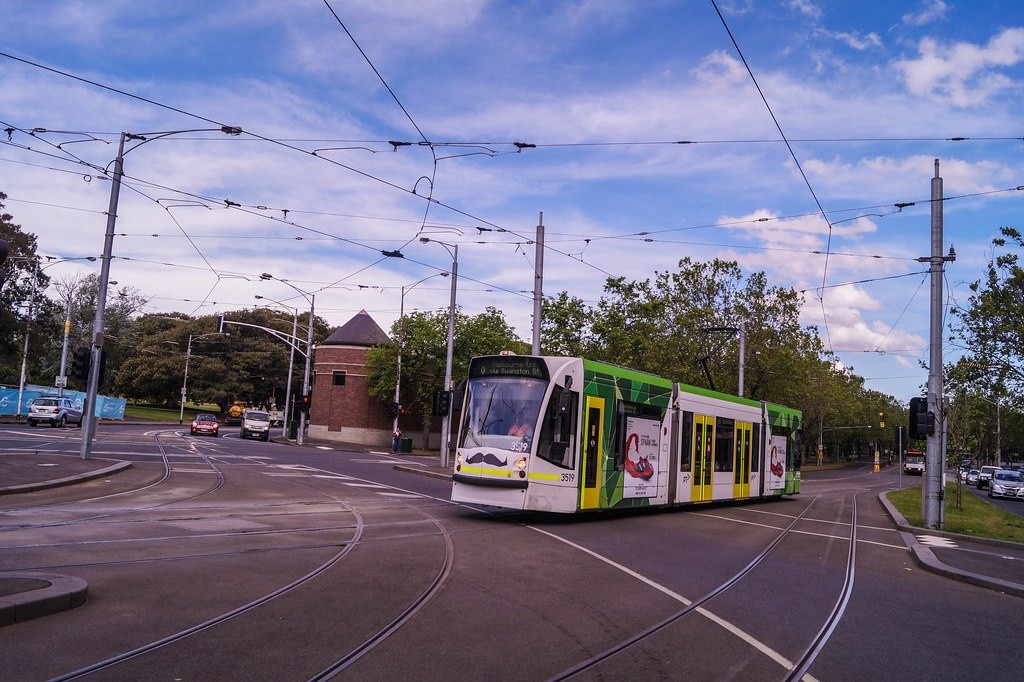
450, 350, 804, 517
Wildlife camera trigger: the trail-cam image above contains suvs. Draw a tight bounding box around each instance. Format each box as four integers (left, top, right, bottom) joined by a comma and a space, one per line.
27, 394, 83, 427
976, 465, 1004, 489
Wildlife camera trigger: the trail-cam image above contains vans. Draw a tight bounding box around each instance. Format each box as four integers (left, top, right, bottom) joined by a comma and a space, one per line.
240, 410, 271, 441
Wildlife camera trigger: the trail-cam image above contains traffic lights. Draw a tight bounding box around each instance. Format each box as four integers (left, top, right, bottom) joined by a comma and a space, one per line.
302, 395, 310, 405
438, 394, 448, 416
387, 403, 398, 417
399, 408, 405, 417
916, 413, 926, 433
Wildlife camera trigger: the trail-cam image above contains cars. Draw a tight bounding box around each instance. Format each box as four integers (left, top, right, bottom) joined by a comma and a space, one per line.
988, 470, 1024, 500
190, 413, 221, 437
957, 458, 980, 485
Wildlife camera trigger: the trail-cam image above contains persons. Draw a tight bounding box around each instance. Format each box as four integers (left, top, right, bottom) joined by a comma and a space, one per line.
391, 428, 403, 454
508, 410, 533, 438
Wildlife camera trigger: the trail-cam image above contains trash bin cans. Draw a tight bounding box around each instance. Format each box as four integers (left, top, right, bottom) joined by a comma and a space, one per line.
401, 438, 412, 453
288, 420, 298, 439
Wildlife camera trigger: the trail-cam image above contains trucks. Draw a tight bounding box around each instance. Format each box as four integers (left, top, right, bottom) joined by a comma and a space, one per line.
904, 450, 926, 476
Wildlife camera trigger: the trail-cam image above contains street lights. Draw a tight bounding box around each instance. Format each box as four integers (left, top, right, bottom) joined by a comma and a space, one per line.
16, 256, 96, 420
179, 333, 231, 424
80, 125, 244, 460
262, 272, 315, 445
391, 270, 451, 452
254, 294, 297, 436
419, 236, 459, 468
